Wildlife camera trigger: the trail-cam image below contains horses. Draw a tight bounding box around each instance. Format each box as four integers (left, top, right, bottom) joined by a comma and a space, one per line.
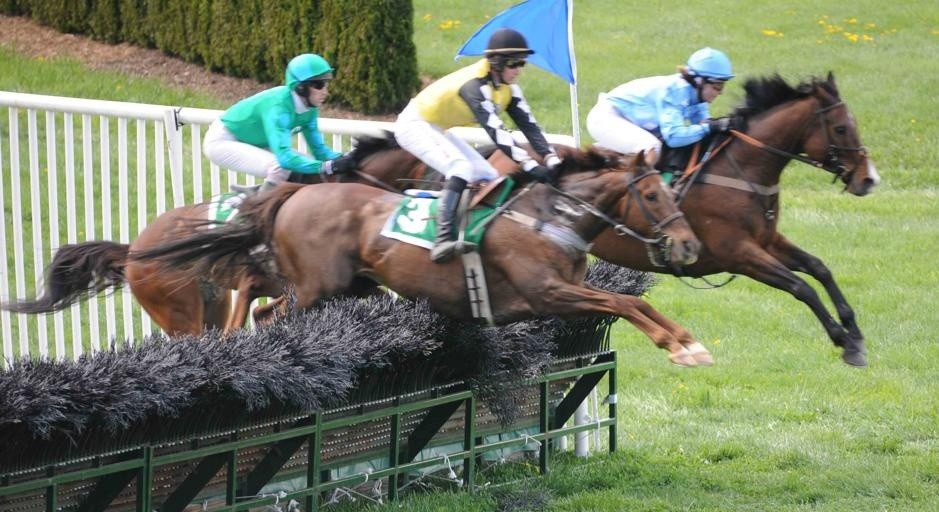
127, 146, 714, 368
1, 128, 421, 337
422, 70, 878, 367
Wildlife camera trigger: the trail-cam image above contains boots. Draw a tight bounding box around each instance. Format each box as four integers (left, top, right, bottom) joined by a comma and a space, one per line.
430, 190, 476, 264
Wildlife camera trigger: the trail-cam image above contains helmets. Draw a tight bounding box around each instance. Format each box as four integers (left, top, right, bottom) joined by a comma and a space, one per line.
285, 54, 336, 89
687, 47, 734, 82
482, 29, 537, 56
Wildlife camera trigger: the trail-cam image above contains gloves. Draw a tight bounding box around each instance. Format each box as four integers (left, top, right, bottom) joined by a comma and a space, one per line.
326, 157, 354, 175
529, 162, 565, 187
709, 116, 747, 133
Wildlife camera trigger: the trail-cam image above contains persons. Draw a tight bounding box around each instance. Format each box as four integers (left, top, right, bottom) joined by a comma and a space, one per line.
202, 53, 360, 197
585, 49, 744, 167
391, 29, 562, 263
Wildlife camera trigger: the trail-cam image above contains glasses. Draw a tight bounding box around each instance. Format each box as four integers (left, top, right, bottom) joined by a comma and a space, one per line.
298, 79, 333, 91
490, 58, 529, 69
704, 81, 728, 92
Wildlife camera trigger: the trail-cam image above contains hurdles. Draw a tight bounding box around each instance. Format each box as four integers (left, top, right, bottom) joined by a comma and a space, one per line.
0, 262, 659, 512
